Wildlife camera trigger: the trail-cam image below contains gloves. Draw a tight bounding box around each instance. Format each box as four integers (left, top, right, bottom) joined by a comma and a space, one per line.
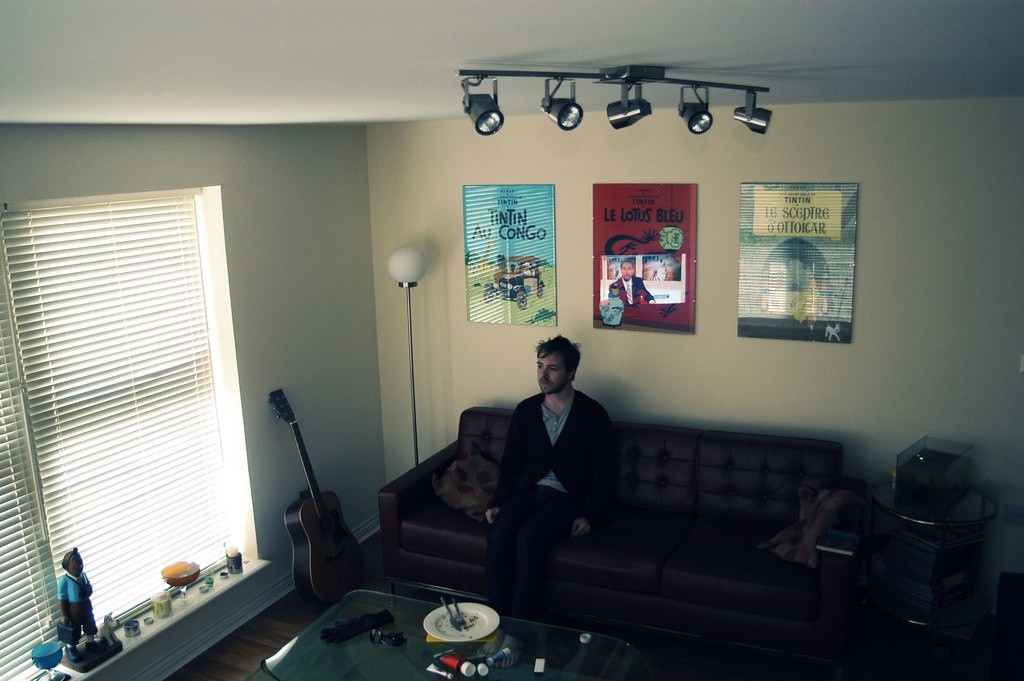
320, 609, 395, 644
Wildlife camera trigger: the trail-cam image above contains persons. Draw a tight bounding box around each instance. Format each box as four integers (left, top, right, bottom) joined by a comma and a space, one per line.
609, 259, 656, 306
58, 547, 104, 662
475, 336, 613, 668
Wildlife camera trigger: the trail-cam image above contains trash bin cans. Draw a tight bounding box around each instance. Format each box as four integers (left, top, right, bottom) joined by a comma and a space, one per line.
989, 571, 1024, 681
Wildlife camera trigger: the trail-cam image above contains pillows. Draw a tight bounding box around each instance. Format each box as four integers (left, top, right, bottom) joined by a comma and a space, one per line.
751, 482, 873, 568
430, 440, 500, 524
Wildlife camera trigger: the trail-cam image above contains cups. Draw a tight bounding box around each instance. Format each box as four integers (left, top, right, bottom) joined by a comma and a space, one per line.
123, 619, 141, 637
226, 552, 243, 573
151, 591, 173, 617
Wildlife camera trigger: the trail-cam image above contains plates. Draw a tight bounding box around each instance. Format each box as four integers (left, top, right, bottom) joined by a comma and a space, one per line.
423, 603, 500, 642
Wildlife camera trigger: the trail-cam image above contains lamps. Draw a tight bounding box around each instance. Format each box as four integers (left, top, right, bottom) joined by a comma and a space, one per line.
388, 244, 427, 465
456, 65, 774, 135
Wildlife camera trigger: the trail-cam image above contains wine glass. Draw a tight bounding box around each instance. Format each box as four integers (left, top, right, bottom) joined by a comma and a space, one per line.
29, 642, 65, 681
160, 561, 200, 610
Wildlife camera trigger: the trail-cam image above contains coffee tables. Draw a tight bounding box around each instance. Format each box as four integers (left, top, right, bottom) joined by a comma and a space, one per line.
245, 588, 654, 681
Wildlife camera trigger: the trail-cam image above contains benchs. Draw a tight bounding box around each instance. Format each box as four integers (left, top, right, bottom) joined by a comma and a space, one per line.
378, 404, 867, 658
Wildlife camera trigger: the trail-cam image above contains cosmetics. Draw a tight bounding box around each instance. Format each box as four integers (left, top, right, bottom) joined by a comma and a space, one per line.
426, 647, 511, 680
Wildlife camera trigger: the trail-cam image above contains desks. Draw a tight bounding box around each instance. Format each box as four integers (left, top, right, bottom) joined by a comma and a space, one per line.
864, 480, 1002, 662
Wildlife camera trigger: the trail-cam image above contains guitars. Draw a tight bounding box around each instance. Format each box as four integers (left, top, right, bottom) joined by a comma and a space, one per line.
268, 388, 366, 606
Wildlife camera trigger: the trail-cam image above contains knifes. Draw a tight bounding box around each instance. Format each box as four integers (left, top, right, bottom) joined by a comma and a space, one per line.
440, 597, 461, 630
